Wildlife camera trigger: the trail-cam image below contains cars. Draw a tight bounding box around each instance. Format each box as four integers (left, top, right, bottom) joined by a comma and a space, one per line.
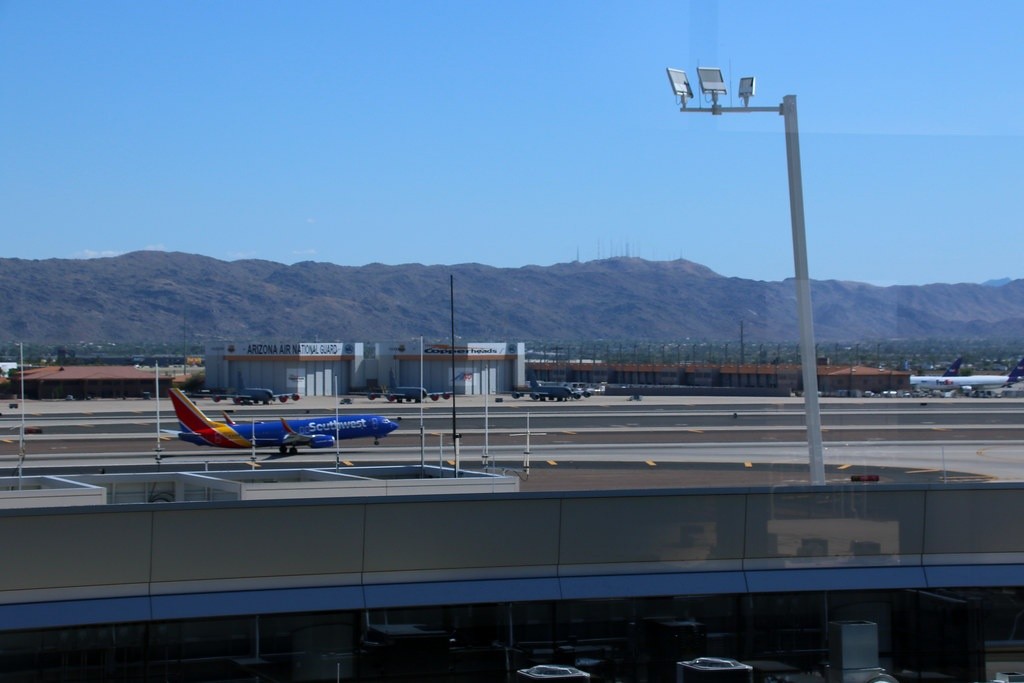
865, 387, 911, 398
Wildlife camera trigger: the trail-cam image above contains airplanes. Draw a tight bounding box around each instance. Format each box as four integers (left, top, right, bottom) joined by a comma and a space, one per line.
184, 387, 301, 406
493, 370, 594, 402
909, 353, 1024, 398
350, 384, 453, 403
157, 388, 401, 457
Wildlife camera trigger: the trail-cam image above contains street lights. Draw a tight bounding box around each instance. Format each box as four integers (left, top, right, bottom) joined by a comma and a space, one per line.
666, 63, 826, 484
14, 341, 25, 490
